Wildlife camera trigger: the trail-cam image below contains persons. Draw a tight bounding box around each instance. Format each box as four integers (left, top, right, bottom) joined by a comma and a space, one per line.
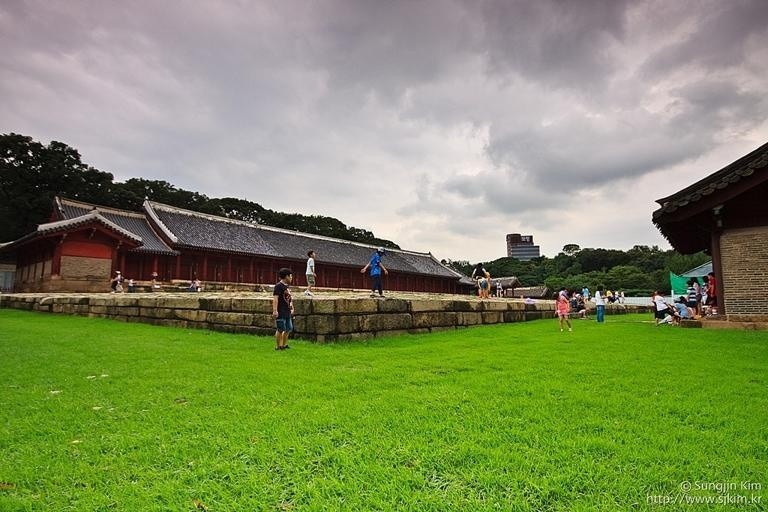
360, 246, 390, 298
303, 250, 318, 296
552, 292, 572, 332
273, 267, 295, 352
651, 271, 717, 327
552, 285, 625, 324
471, 263, 504, 299
109, 270, 203, 294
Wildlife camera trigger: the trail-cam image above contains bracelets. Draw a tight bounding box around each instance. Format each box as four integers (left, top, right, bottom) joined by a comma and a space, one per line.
383, 268, 386, 271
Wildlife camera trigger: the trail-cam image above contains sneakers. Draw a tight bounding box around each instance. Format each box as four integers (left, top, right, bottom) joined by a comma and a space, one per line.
275, 344, 290, 351
304, 289, 315, 298
369, 292, 386, 297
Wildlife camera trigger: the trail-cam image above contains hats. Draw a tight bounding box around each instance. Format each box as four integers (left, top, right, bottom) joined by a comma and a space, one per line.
377, 247, 387, 257
